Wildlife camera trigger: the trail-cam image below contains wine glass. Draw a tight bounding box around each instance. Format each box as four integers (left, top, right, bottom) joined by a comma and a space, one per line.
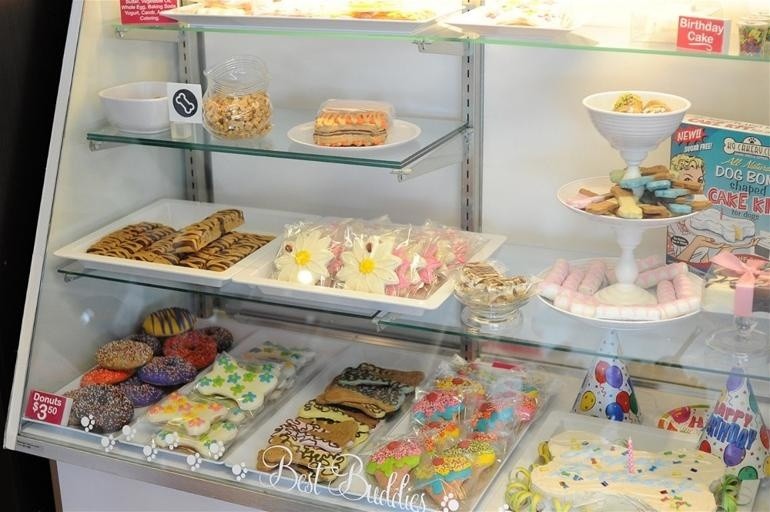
581, 92, 691, 192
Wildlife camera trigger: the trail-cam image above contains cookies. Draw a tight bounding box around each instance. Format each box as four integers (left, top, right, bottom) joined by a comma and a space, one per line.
146, 339, 317, 461
569, 164, 710, 220
255, 362, 422, 485
368, 368, 543, 512
275, 230, 453, 295
689, 209, 755, 242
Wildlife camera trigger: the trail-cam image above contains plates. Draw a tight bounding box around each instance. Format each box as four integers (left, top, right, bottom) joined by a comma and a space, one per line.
25, 315, 256, 440
556, 177, 707, 226
227, 341, 439, 489
535, 258, 705, 326
343, 354, 553, 512
117, 326, 353, 465
287, 116, 421, 156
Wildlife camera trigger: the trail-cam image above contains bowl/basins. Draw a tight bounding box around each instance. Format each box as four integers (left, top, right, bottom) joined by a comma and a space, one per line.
98, 80, 183, 135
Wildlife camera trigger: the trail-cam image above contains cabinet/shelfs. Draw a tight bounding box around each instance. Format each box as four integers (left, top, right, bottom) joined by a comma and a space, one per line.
3, 1, 770, 512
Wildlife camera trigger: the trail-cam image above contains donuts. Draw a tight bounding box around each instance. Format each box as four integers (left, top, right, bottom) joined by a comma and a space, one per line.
60, 306, 233, 434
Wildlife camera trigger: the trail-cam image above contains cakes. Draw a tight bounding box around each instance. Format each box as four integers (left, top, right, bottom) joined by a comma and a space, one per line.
505, 429, 741, 512
313, 99, 390, 148
87, 208, 273, 274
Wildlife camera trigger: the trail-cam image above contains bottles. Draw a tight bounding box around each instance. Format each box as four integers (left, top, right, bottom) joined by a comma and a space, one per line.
197, 54, 274, 138
